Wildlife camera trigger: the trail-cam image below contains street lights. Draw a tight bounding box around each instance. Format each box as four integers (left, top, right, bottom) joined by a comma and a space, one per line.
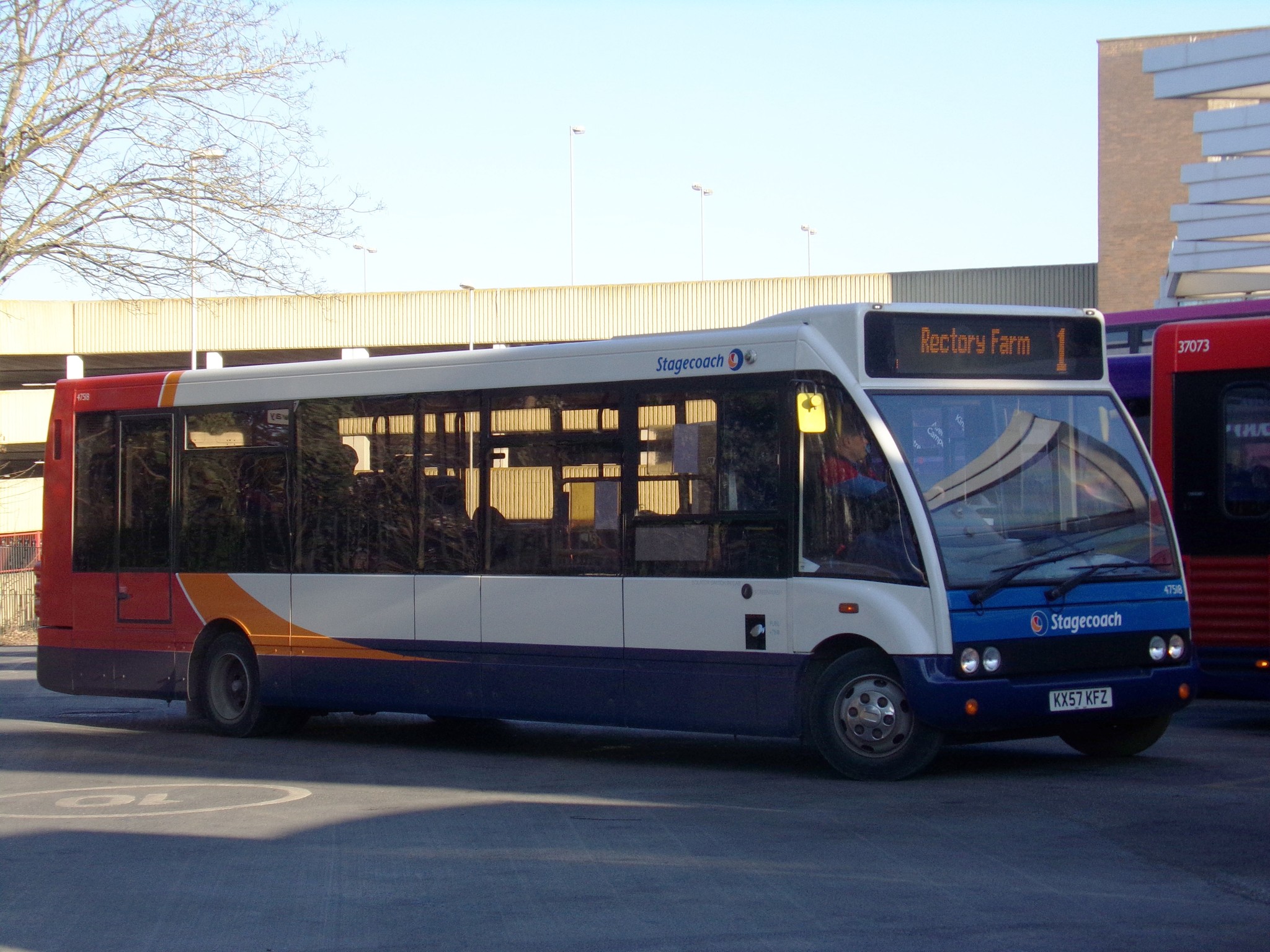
186, 149, 226, 370
798, 223, 818, 273
566, 124, 589, 289
692, 184, 712, 282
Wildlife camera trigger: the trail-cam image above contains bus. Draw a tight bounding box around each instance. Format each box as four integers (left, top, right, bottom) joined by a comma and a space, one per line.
37, 300, 1200, 784
1147, 315, 1270, 650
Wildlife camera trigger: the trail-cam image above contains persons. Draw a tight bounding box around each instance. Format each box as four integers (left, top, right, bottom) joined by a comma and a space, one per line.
823, 429, 896, 541
